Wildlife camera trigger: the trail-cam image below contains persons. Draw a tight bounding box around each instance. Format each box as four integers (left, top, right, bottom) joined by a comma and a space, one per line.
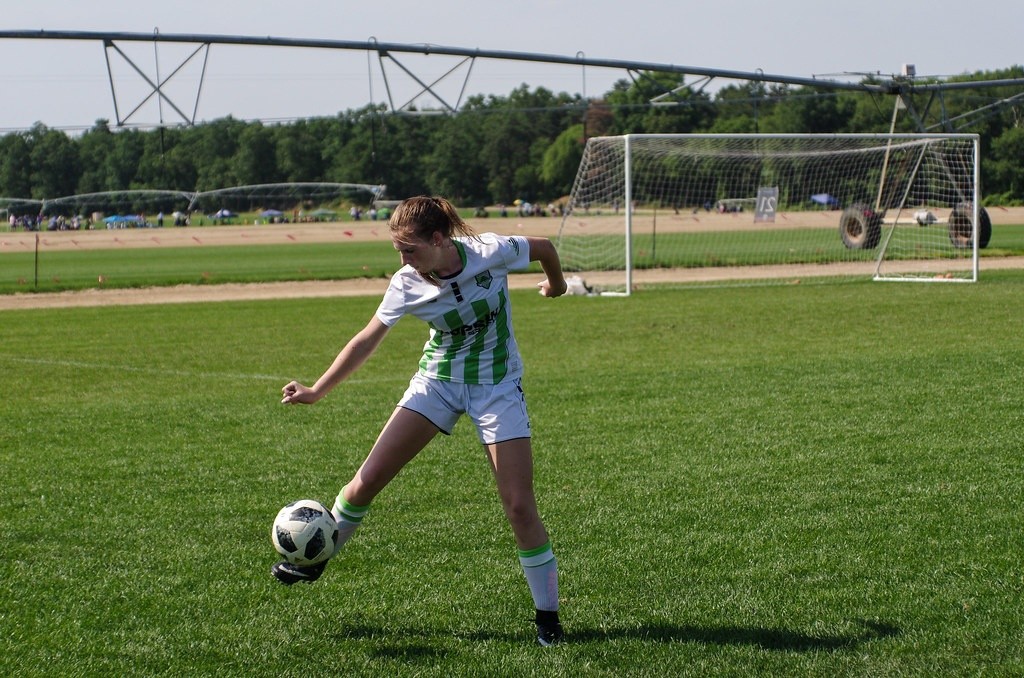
272, 197, 568, 647
85, 217, 93, 230
350, 204, 391, 221
500, 204, 507, 217
473, 206, 489, 217
515, 201, 564, 216
182, 214, 188, 226
106, 222, 126, 229
157, 211, 163, 227
583, 197, 590, 211
614, 199, 618, 212
10, 214, 81, 231
172, 208, 180, 225
254, 216, 289, 224
704, 200, 743, 213
292, 216, 325, 223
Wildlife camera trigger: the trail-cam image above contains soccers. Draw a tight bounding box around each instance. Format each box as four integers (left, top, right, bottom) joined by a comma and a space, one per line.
271, 499, 339, 568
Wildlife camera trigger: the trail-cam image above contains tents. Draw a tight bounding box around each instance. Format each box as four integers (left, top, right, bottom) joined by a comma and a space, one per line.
811, 193, 839, 205
311, 207, 336, 215
103, 215, 128, 222
260, 209, 284, 217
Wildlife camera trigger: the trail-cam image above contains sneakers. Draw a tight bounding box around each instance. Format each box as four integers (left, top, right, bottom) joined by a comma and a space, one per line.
535, 621, 566, 646
271, 562, 328, 585
